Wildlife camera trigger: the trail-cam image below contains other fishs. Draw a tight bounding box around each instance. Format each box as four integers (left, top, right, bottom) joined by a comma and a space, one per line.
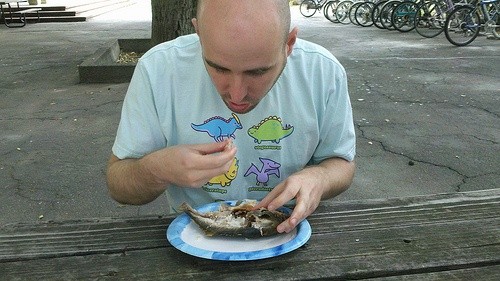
176, 198, 292, 240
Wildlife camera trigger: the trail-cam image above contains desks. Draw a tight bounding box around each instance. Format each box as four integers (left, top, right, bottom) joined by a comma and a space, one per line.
1, 188, 500, 281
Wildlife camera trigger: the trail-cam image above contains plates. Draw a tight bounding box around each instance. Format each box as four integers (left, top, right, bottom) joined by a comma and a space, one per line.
167, 200, 311, 261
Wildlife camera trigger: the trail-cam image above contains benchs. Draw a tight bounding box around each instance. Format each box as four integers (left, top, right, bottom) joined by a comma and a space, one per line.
1, 2, 42, 28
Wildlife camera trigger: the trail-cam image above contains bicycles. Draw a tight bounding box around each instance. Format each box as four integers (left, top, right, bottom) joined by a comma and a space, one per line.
299, 0, 500, 46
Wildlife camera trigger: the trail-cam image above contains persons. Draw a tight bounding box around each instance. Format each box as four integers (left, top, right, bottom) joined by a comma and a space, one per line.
106, 0, 357, 234
